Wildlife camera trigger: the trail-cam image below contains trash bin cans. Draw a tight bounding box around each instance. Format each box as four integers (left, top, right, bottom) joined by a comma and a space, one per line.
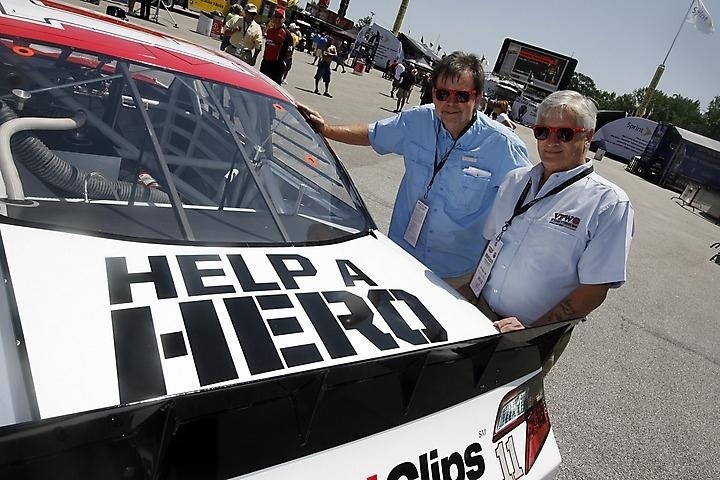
352, 57, 366, 76
594, 146, 607, 161
209, 11, 227, 41
195, 10, 215, 38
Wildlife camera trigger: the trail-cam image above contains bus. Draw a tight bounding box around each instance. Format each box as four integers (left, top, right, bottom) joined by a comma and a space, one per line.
157, 0, 298, 27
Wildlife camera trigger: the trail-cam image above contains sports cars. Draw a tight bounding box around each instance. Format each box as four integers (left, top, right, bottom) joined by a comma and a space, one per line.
0, 0, 583, 480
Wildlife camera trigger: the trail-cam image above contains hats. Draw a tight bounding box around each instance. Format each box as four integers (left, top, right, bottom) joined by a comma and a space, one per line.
275, 7, 286, 18
244, 3, 258, 13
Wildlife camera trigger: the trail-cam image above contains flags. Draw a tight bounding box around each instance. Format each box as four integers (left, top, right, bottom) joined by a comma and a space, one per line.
682, 0, 715, 35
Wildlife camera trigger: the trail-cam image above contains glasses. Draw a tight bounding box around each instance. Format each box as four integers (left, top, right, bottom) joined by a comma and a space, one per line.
435, 88, 476, 103
532, 125, 587, 143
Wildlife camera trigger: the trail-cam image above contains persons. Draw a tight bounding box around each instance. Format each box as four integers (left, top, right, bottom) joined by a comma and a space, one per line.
282, 23, 337, 98
220, 4, 293, 85
128, 1, 150, 21
478, 91, 517, 129
382, 59, 433, 112
294, 49, 535, 290
331, 40, 349, 73
459, 88, 637, 377
